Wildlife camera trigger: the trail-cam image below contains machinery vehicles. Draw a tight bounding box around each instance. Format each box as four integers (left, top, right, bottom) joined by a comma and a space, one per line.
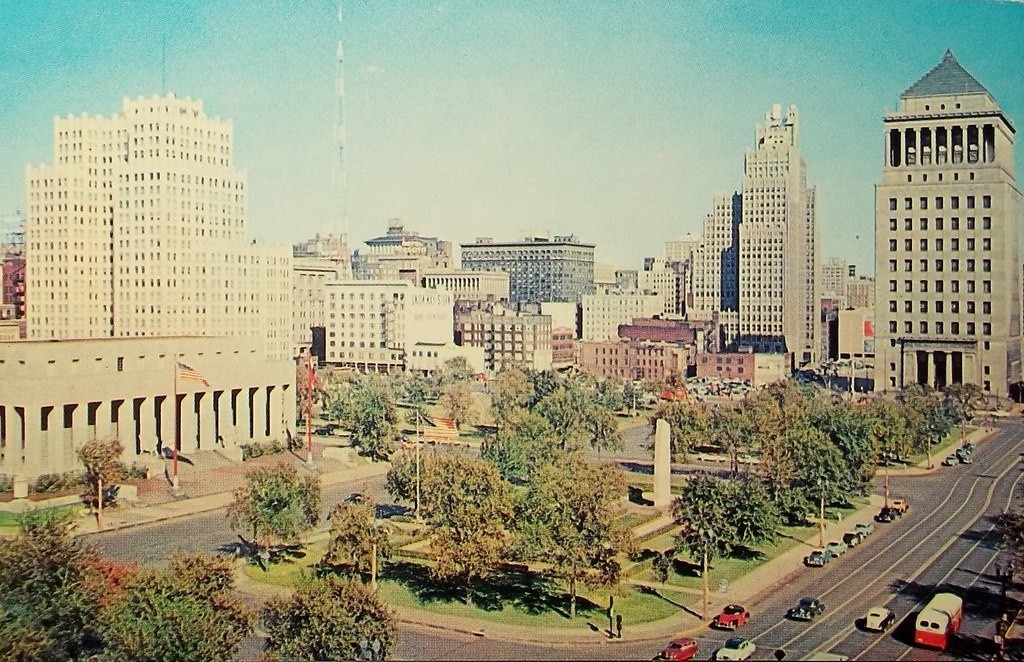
956, 443, 973, 464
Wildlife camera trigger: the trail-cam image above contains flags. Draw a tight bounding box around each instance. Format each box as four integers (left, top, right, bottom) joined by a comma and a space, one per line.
421, 416, 459, 443
177, 362, 210, 387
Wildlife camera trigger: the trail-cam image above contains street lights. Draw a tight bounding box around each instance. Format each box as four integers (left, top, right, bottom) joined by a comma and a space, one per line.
698, 527, 713, 622
816, 477, 829, 549
882, 443, 892, 509
923, 424, 935, 469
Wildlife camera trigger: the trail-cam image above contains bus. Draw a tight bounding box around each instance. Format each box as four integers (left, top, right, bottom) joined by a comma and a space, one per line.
914, 593, 963, 649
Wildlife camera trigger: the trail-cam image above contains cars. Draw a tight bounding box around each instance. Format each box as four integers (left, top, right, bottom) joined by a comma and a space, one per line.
841, 531, 864, 547
826, 540, 847, 558
855, 608, 896, 634
723, 382, 749, 395
942, 456, 960, 466
874, 506, 902, 523
712, 603, 751, 631
716, 637, 756, 662
855, 522, 874, 537
784, 597, 825, 623
656, 638, 698, 662
806, 549, 832, 567
315, 424, 343, 436
889, 500, 909, 514
